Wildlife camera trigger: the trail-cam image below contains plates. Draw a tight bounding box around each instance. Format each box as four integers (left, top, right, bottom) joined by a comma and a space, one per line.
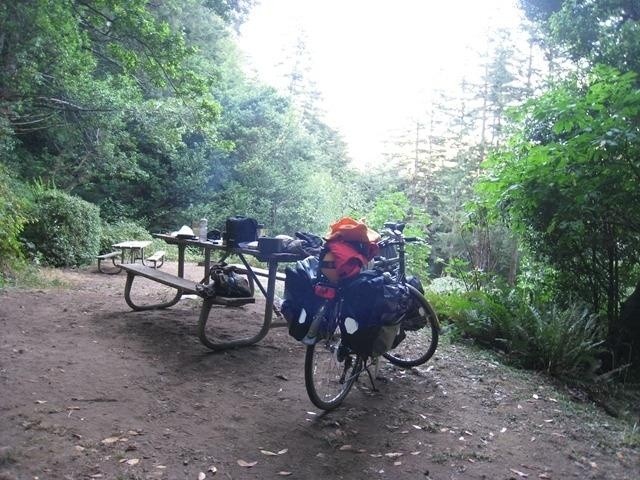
238, 241, 260, 249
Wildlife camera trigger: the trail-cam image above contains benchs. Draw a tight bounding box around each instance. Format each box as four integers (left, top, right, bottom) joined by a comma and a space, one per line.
140, 249, 166, 269
96, 251, 121, 274
227, 263, 286, 281
117, 262, 254, 351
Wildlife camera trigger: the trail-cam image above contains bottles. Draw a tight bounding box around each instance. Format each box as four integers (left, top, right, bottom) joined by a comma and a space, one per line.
255, 223, 265, 240
192, 217, 209, 239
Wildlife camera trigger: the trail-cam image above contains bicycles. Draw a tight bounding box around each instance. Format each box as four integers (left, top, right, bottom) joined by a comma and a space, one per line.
303, 221, 444, 410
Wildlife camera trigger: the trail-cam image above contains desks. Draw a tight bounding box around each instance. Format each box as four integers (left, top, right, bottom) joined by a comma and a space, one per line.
111, 240, 154, 272
152, 229, 309, 345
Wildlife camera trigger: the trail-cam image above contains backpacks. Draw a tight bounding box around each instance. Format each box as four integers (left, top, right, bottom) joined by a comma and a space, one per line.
340, 269, 388, 349
281, 256, 329, 341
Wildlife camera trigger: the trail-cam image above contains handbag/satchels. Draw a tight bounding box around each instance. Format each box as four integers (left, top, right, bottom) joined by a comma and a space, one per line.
226, 214, 260, 248
210, 261, 251, 297
